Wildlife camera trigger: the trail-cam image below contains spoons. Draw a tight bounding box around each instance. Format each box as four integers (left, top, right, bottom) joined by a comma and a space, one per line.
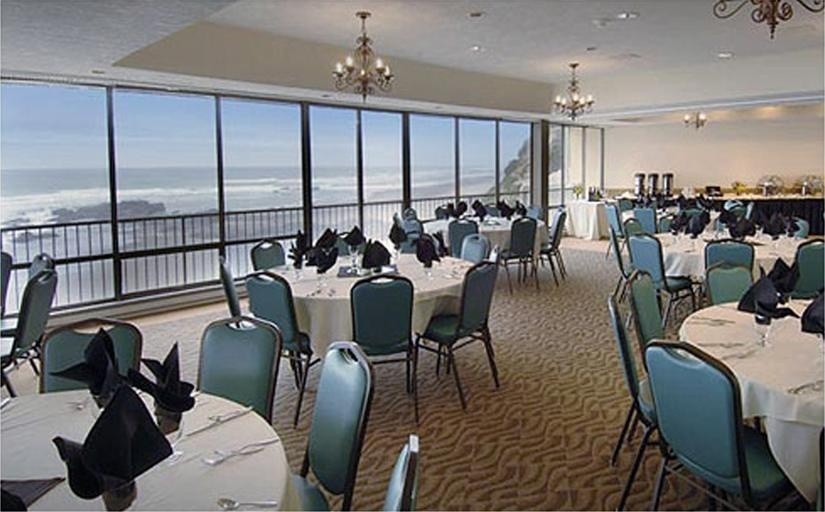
216, 498, 278, 509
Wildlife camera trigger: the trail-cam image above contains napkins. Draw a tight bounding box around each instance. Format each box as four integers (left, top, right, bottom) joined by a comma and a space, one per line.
126, 340, 196, 432
50, 387, 173, 512
49, 326, 130, 406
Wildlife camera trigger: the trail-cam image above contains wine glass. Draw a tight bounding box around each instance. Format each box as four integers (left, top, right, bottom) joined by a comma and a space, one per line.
290, 246, 447, 295
89, 391, 185, 511
668, 220, 795, 255
751, 294, 825, 371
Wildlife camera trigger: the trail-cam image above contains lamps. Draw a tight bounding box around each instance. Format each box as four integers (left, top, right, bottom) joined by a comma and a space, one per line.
550, 60, 593, 123
333, 11, 397, 100
712, 0, 824, 43
681, 111, 709, 132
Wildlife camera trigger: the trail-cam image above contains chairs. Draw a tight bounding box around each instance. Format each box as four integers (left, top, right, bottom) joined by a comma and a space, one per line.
383, 433, 421, 512
215, 199, 568, 422
292, 339, 375, 512
1, 251, 58, 377
604, 178, 824, 511
197, 316, 283, 427
39, 315, 144, 394
2, 269, 57, 399
